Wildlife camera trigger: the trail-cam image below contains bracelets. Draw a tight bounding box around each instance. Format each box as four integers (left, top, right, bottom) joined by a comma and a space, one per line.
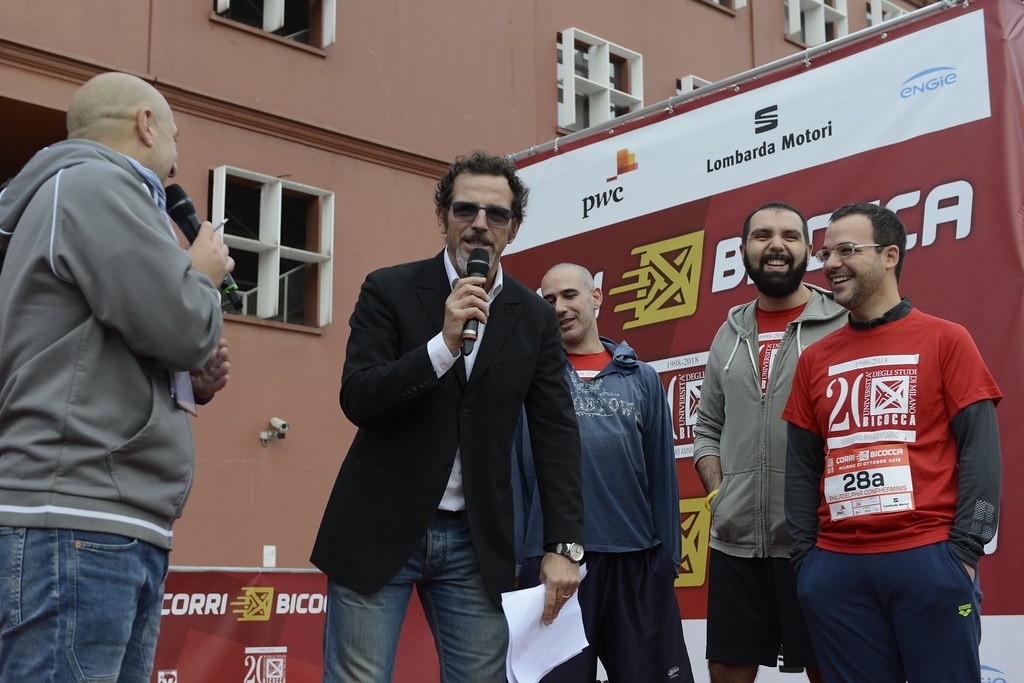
704, 489, 719, 512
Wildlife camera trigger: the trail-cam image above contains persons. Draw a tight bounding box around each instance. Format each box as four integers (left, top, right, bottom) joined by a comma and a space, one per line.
308, 150, 587, 683
783, 202, 1003, 683
1, 70, 236, 683
513, 262, 696, 683
693, 201, 848, 683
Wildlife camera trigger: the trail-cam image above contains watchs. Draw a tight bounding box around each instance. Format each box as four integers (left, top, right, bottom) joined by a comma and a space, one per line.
556, 542, 584, 563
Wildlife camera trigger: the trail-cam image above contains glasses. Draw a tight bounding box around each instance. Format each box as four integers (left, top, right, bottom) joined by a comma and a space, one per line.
814, 242, 888, 262
450, 201, 514, 225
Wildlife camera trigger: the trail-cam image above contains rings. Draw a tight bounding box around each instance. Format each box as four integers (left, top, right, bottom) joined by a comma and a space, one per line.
563, 595, 570, 598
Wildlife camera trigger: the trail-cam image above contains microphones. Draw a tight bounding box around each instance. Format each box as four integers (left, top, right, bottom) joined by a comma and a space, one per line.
166, 183, 243, 311
462, 248, 490, 356
